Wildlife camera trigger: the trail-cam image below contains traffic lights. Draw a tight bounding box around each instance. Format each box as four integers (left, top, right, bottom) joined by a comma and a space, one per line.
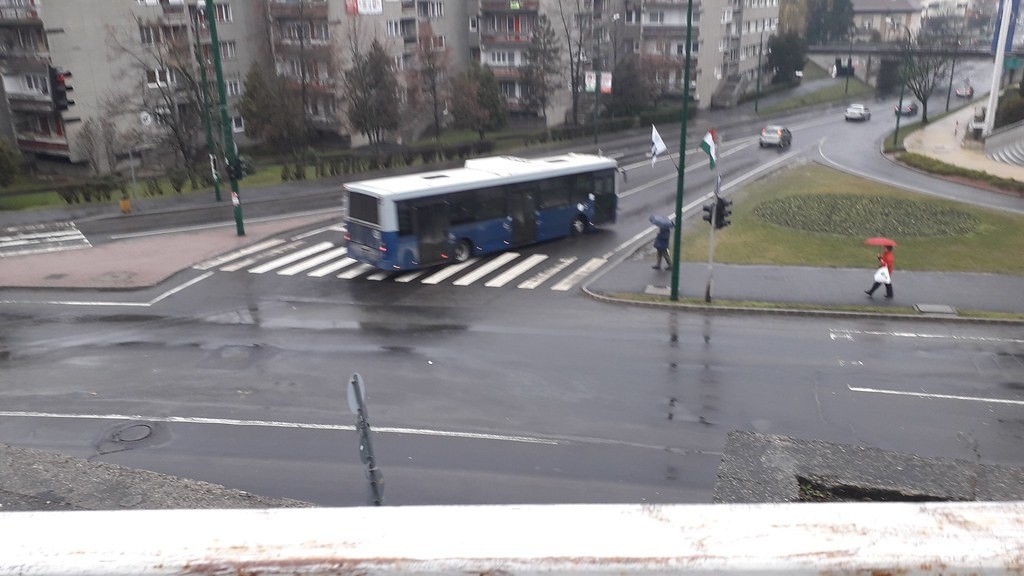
703, 203, 715, 225
45, 62, 75, 113
714, 194, 734, 230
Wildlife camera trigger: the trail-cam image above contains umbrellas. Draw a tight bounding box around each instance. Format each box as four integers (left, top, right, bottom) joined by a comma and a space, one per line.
863, 237, 897, 261
649, 212, 675, 229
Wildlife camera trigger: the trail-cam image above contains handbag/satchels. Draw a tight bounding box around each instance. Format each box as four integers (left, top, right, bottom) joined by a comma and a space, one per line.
874, 265, 890, 284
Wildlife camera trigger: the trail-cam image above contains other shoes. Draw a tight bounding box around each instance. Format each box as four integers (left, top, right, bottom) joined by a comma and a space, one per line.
652, 265, 673, 271
864, 289, 893, 298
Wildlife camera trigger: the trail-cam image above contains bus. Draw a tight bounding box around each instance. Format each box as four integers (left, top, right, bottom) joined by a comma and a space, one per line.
341, 151, 631, 276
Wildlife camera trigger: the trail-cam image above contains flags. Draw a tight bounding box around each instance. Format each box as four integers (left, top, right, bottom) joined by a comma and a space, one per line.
698, 127, 719, 171
646, 125, 667, 169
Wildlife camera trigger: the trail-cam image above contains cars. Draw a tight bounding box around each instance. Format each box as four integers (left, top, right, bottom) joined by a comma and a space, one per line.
956, 85, 975, 98
894, 99, 919, 116
844, 103, 871, 122
760, 125, 793, 149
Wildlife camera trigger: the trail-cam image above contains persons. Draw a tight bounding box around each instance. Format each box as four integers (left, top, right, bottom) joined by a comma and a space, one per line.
648, 214, 673, 271
863, 246, 895, 299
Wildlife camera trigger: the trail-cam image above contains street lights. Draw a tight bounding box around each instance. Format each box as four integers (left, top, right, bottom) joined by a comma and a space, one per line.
885, 15, 912, 145
592, 12, 620, 146
191, 1, 227, 205
